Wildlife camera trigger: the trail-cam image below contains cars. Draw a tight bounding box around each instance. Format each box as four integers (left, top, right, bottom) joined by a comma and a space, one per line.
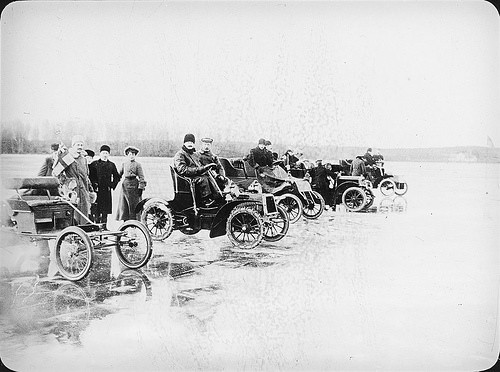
228, 154, 408, 224
0, 177, 154, 282
135, 157, 290, 250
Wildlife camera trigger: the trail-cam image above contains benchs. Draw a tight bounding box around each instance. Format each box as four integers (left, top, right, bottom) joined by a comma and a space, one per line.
6, 176, 70, 207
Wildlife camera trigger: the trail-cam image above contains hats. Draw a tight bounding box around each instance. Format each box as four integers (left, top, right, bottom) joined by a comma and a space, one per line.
71, 135, 84, 146
124, 147, 139, 156
258, 139, 265, 144
100, 145, 110, 154
85, 149, 95, 158
201, 138, 213, 143
183, 133, 196, 143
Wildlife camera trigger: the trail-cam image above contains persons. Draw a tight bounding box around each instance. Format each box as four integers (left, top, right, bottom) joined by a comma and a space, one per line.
199, 138, 225, 181
249, 139, 273, 170
315, 160, 335, 210
116, 146, 146, 222
39, 144, 64, 177
174, 133, 216, 207
364, 148, 373, 160
284, 150, 303, 163
84, 150, 95, 164
88, 145, 120, 230
62, 135, 92, 223
350, 156, 365, 176
